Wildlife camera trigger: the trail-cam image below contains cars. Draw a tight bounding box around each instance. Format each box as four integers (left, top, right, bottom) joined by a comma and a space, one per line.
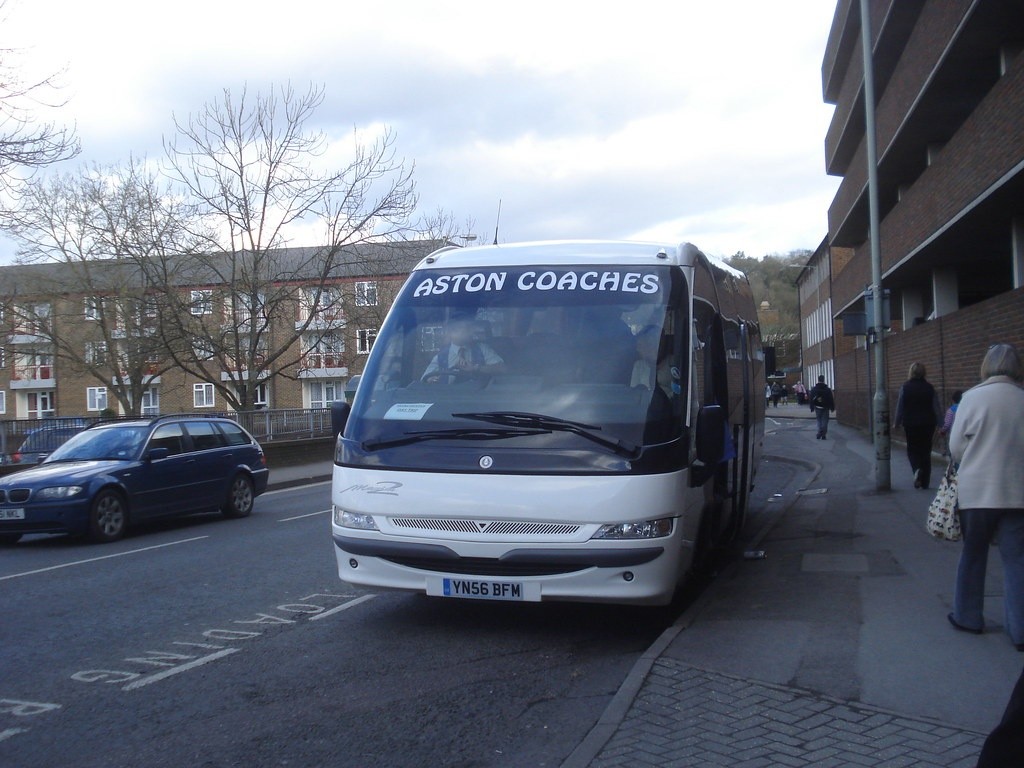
2, 415, 270, 543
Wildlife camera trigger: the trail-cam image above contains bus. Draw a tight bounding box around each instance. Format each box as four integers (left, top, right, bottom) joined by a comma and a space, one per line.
334, 238, 768, 613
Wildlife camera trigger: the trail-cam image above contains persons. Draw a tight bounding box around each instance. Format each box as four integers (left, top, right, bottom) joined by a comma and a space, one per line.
771, 381, 781, 408
630, 325, 676, 397
420, 314, 506, 384
943, 343, 1024, 651
766, 382, 771, 407
938, 390, 963, 436
891, 362, 944, 489
810, 374, 834, 440
780, 384, 790, 404
792, 380, 807, 405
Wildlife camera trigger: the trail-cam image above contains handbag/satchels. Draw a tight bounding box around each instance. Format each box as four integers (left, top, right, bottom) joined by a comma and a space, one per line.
926, 458, 961, 542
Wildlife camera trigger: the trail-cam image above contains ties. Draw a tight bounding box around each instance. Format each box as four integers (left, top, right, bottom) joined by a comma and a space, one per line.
455, 348, 469, 383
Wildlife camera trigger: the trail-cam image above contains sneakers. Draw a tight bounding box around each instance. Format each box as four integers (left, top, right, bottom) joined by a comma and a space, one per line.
913, 468, 924, 489
817, 433, 826, 440
1015, 643, 1024, 652
921, 484, 930, 488
948, 613, 983, 633
